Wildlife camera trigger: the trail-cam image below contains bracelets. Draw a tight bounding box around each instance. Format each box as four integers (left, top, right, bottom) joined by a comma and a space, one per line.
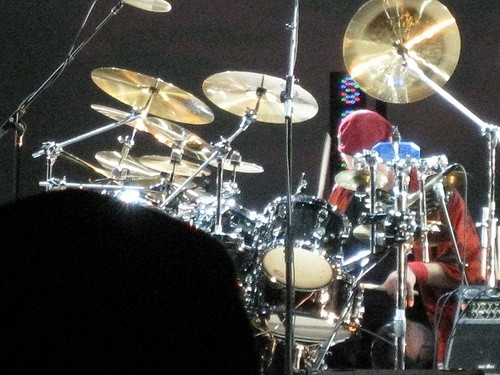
409, 262, 428, 284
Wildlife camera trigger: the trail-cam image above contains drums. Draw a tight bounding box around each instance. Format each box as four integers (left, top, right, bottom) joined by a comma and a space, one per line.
242, 263, 366, 346
258, 193, 351, 291
191, 195, 264, 279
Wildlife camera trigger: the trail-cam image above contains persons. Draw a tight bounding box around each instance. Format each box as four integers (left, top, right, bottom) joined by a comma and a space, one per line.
329, 109, 482, 369
0, 187, 262, 375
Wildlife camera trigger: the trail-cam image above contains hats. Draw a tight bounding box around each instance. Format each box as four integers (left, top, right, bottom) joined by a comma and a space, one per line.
336, 109, 393, 154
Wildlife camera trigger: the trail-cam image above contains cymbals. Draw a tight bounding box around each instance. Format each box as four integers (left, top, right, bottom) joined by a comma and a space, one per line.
144, 117, 213, 155
140, 191, 165, 204
138, 154, 211, 177
206, 158, 265, 174
202, 71, 319, 124
352, 218, 458, 248
425, 171, 474, 188
91, 103, 150, 133
342, 0, 461, 104
94, 151, 207, 200
91, 67, 215, 125
123, 0, 172, 13
334, 168, 390, 192
